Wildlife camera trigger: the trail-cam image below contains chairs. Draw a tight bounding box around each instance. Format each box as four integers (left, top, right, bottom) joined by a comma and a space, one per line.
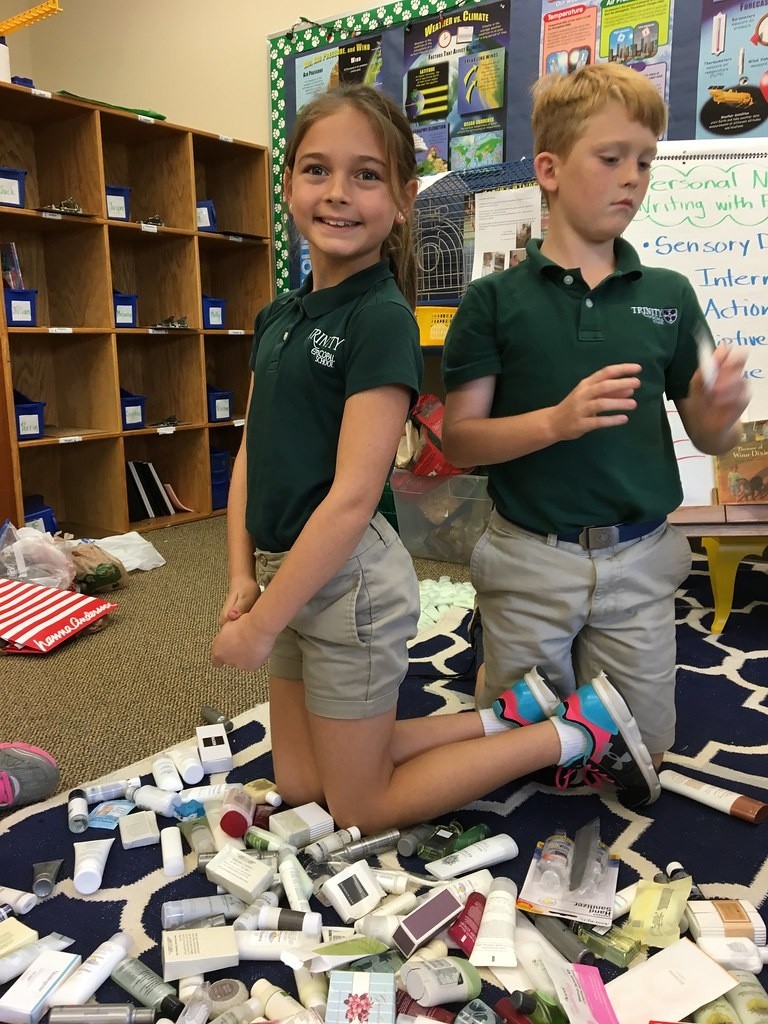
702, 419, 768, 634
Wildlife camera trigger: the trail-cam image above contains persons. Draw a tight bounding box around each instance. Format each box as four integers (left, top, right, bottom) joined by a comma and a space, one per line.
482, 220, 535, 286
0, 741, 59, 809
207, 83, 665, 835
441, 62, 752, 775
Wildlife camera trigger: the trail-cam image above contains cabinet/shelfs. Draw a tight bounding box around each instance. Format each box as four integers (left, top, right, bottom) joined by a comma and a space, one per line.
0, 81, 275, 540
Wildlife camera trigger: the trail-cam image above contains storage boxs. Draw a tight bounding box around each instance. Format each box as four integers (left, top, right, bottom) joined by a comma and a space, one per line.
389, 468, 493, 564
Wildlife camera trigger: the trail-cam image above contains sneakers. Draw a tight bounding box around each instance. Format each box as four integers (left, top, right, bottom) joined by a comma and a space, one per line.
490, 665, 565, 725
2, 743, 63, 809
552, 673, 661, 812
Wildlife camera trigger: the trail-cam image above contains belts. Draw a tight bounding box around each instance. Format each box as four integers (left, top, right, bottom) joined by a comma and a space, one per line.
494, 503, 681, 551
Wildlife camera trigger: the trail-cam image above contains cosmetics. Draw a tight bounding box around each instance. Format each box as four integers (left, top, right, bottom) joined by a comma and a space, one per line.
0, 705, 768, 1024
658, 770, 768, 824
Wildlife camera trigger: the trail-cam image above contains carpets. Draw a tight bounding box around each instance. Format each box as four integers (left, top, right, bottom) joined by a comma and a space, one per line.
0, 549, 768, 1024
